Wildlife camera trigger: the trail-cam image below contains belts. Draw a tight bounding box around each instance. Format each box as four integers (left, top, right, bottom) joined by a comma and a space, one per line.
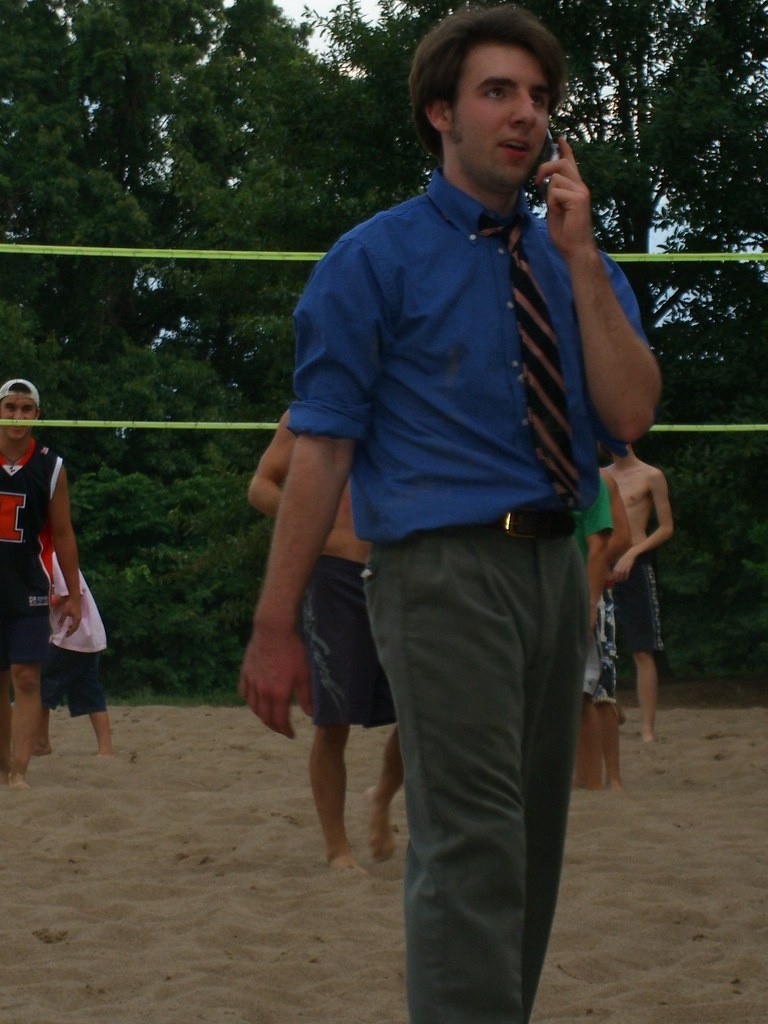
429, 505, 577, 542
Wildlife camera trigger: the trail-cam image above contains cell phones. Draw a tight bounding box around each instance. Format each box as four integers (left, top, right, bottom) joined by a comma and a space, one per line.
534, 126, 560, 194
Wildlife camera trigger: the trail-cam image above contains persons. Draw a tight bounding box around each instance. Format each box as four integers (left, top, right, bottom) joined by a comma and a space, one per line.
249, 408, 404, 866
239, 7, 663, 1023
33, 552, 111, 756
574, 447, 675, 791
0, 379, 84, 788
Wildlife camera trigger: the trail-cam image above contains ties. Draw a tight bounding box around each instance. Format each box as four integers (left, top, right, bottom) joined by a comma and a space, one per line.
482, 211, 580, 509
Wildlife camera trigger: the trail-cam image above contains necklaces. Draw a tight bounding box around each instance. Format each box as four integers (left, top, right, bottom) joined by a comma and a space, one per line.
1, 452, 25, 473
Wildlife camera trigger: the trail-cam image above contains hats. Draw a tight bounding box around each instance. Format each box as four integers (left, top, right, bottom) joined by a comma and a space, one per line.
0, 379, 40, 408
